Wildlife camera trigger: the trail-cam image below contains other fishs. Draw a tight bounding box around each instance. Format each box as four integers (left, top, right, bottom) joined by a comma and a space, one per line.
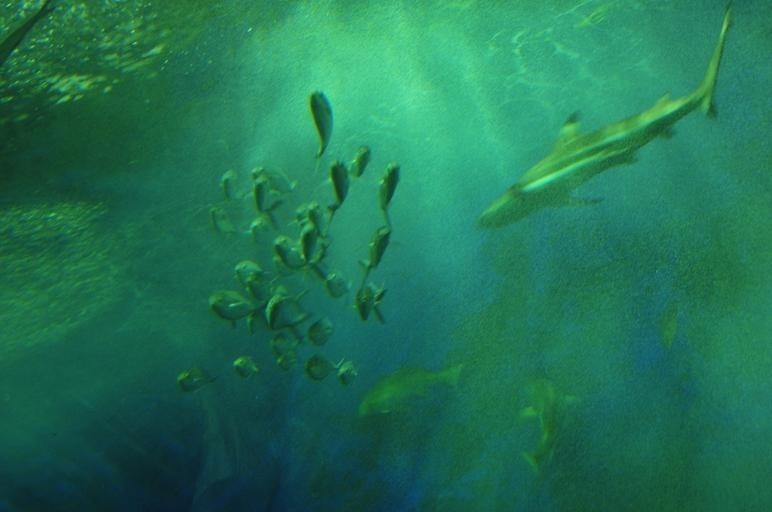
208, 148, 399, 383
